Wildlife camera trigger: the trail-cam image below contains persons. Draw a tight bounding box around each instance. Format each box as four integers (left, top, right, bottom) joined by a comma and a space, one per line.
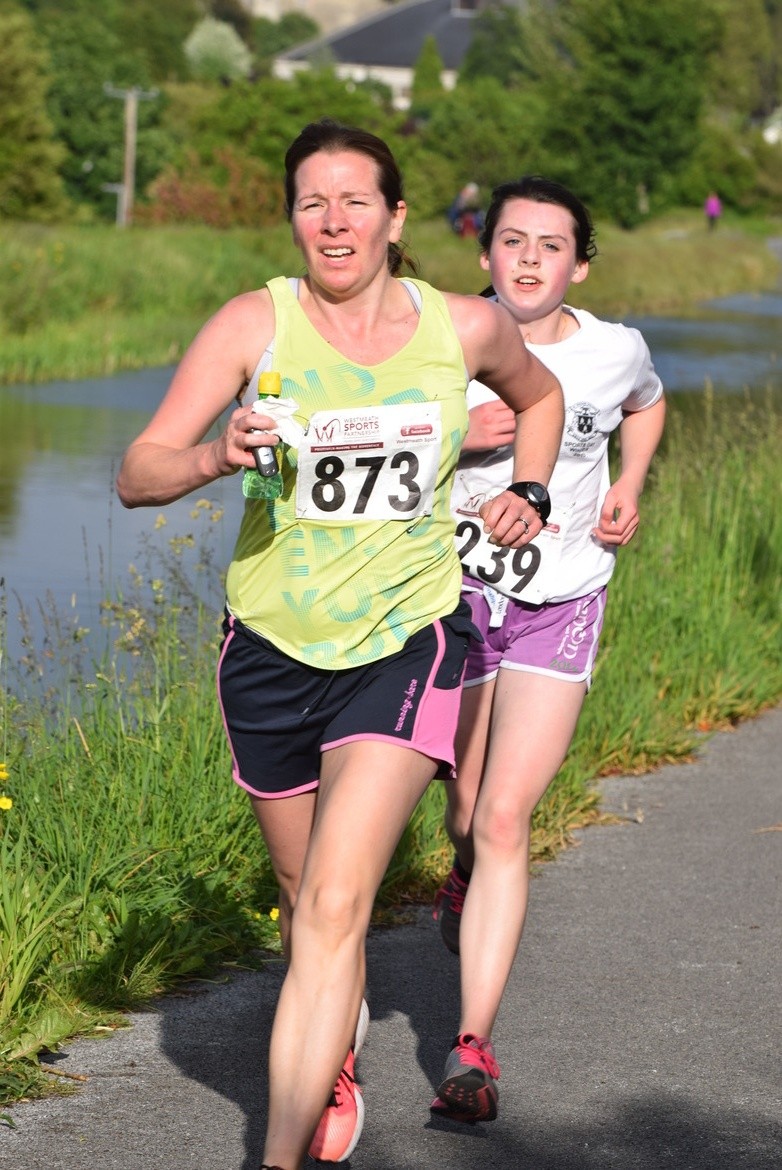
116, 124, 566, 1170
429, 179, 666, 1121
704, 188, 720, 233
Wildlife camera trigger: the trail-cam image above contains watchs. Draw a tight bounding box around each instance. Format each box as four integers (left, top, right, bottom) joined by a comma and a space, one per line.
507, 481, 551, 524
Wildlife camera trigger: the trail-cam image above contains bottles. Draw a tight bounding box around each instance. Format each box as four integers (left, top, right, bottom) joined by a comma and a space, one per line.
242, 371, 285, 501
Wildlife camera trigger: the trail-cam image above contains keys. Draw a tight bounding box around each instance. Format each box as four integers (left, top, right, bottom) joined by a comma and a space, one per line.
247, 429, 279, 478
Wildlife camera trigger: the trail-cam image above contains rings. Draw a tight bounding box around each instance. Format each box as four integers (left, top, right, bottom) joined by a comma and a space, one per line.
517, 518, 528, 533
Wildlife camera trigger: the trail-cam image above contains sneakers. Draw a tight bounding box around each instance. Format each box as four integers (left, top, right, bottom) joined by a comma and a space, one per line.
430, 1034, 500, 1125
433, 852, 471, 954
301, 994, 370, 1163
